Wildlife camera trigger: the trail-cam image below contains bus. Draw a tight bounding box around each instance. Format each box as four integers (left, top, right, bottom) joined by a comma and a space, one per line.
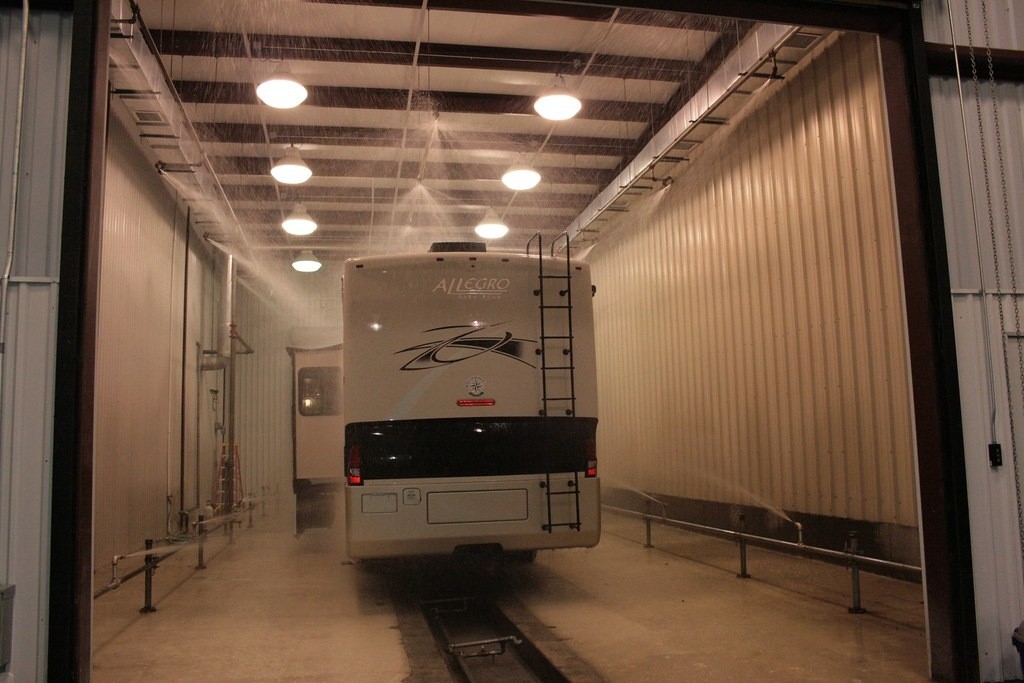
340, 233, 604, 561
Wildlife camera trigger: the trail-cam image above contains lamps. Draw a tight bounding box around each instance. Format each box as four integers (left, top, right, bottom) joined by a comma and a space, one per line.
292, 250, 322, 272
256, 60, 307, 109
475, 207, 510, 240
502, 154, 541, 190
270, 146, 313, 184
534, 73, 582, 120
281, 204, 318, 235
